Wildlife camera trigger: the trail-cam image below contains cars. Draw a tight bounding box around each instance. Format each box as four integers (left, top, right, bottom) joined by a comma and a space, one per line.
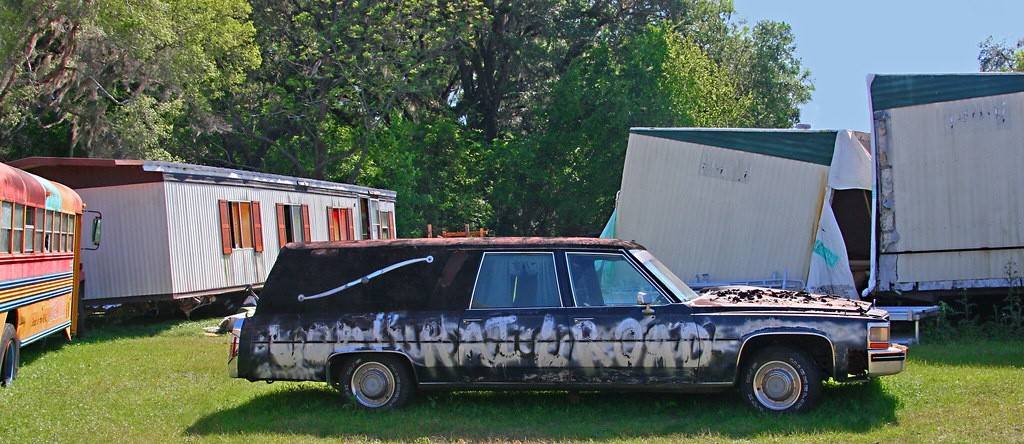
228, 233, 909, 419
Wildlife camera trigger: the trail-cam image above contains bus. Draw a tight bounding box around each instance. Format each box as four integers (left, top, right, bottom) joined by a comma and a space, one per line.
0, 163, 103, 391
